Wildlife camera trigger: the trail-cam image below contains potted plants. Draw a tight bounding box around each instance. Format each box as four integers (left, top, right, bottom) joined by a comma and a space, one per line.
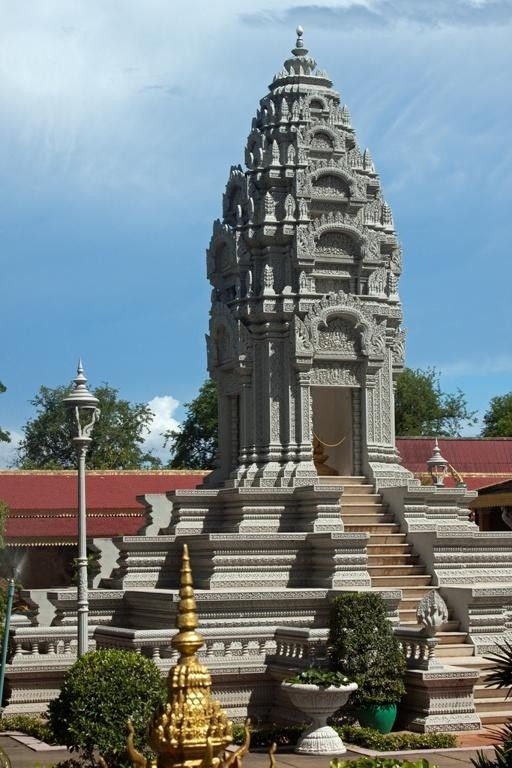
342, 628, 407, 736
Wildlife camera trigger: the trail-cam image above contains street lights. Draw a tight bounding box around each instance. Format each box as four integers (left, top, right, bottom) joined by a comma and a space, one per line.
425, 436, 448, 487
60, 358, 102, 664
